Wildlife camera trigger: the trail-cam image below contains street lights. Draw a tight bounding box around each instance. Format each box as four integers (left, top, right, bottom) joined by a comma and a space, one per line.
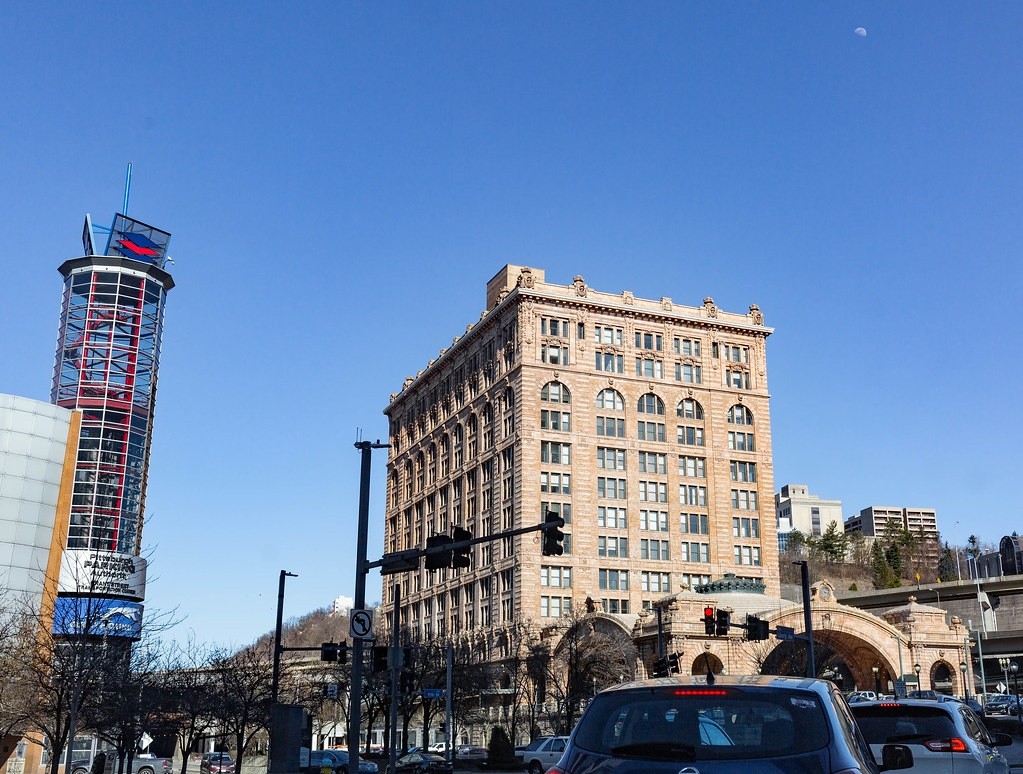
872, 661, 967, 703
999, 658, 1022, 723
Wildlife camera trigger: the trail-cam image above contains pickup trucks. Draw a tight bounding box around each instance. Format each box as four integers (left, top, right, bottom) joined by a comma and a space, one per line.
70, 749, 173, 774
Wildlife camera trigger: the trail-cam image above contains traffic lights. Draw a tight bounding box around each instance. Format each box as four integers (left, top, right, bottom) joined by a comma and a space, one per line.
400, 670, 415, 694
653, 653, 681, 678
453, 511, 565, 567
704, 608, 759, 641
338, 642, 346, 664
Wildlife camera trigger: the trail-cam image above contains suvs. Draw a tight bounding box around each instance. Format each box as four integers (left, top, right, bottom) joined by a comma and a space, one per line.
200, 752, 236, 774
843, 694, 1013, 774
515, 735, 571, 774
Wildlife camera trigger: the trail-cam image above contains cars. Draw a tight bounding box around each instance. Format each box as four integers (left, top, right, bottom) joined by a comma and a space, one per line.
850, 690, 940, 701
660, 711, 737, 746
311, 750, 378, 774
960, 695, 1023, 716
385, 753, 454, 774
544, 675, 914, 774
358, 742, 453, 753
455, 745, 489, 764
